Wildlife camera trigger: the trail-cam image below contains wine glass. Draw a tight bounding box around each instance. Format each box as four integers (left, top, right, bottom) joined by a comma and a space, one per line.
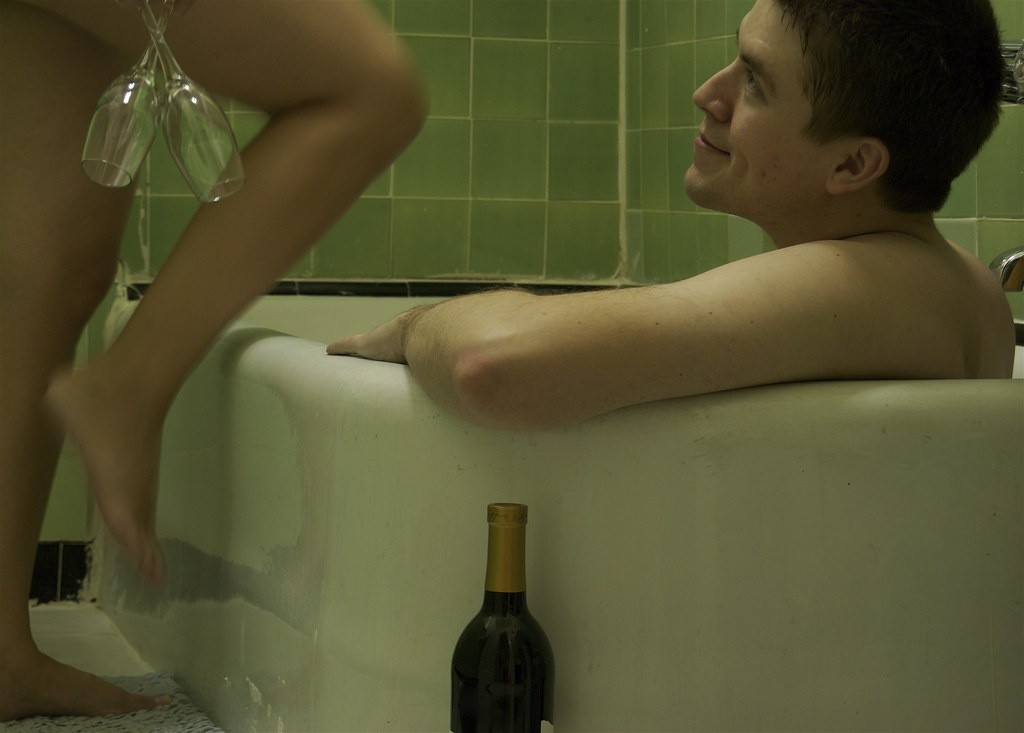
81, 0, 173, 187
137, 0, 244, 201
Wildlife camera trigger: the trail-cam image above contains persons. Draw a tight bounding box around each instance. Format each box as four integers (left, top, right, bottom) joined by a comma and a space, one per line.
326, 0, 1014, 430
0, 0, 431, 721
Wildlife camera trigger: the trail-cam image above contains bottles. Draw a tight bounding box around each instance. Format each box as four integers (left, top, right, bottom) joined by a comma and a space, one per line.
450, 503, 555, 733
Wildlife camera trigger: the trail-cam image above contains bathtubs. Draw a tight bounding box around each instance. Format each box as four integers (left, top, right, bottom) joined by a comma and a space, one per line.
87, 294, 1022, 731
